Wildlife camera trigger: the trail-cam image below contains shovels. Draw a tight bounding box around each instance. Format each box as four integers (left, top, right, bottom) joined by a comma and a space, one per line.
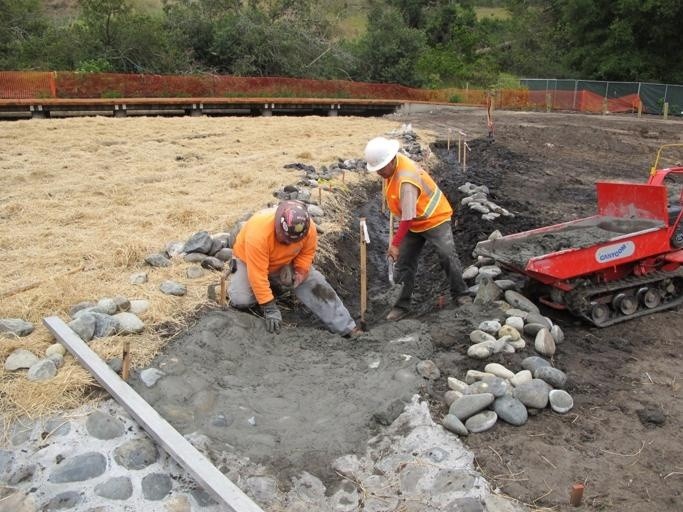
387, 212, 403, 307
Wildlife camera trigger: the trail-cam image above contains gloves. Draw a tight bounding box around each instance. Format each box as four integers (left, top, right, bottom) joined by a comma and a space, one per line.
261, 301, 282, 336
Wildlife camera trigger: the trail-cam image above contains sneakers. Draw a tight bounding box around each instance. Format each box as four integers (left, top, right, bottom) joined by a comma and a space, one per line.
456, 296, 472, 306
349, 327, 363, 338
384, 308, 409, 322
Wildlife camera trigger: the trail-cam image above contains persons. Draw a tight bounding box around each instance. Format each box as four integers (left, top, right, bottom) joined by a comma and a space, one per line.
226, 200, 366, 342
364, 135, 470, 321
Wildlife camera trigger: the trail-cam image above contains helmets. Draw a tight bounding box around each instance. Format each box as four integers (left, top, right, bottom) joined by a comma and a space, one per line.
364, 136, 398, 172
273, 199, 310, 245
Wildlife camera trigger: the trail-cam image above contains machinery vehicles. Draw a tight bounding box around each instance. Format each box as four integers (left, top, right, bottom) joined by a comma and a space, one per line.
475, 164, 682, 329
